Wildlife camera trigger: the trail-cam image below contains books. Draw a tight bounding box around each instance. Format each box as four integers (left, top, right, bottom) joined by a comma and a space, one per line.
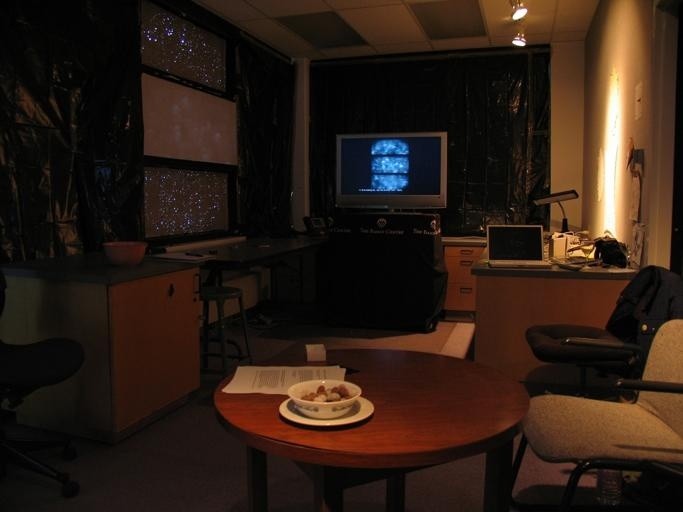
152, 250, 216, 262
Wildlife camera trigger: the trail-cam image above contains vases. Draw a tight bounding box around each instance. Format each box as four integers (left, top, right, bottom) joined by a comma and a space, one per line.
102, 235, 152, 268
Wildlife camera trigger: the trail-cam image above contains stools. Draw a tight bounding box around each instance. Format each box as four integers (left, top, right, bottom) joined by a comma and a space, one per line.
196, 285, 250, 371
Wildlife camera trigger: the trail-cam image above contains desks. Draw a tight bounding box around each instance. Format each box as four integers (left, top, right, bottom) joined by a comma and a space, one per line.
187, 236, 315, 321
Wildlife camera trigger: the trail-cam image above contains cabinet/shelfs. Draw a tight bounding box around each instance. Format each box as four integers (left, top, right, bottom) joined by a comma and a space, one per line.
440, 235, 492, 317
0, 260, 200, 444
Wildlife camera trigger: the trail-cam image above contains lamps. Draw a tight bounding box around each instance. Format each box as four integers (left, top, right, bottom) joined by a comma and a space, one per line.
533, 187, 581, 233
510, 24, 528, 48
506, 0, 529, 22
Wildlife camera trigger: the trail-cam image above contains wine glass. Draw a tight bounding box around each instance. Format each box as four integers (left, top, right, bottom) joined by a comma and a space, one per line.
580, 240, 595, 268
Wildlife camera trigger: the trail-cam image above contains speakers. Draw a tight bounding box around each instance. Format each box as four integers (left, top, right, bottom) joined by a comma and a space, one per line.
549, 238, 566, 260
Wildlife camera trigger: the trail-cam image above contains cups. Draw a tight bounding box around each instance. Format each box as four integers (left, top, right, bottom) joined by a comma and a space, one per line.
595, 467, 622, 506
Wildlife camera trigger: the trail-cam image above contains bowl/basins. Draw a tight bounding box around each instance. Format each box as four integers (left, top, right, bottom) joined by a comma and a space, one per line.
285, 378, 362, 420
101, 242, 148, 266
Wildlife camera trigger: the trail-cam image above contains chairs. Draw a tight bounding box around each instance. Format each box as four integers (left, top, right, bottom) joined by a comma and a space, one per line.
523, 262, 674, 400
505, 316, 683, 510
0, 334, 92, 497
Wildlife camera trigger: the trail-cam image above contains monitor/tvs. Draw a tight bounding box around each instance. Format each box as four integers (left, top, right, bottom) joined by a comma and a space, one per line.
335, 131, 448, 209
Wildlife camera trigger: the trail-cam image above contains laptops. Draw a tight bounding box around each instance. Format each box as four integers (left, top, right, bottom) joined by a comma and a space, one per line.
486, 224, 551, 268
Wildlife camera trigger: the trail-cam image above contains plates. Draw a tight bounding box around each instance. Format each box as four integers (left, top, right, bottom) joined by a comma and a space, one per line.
277, 398, 376, 427
